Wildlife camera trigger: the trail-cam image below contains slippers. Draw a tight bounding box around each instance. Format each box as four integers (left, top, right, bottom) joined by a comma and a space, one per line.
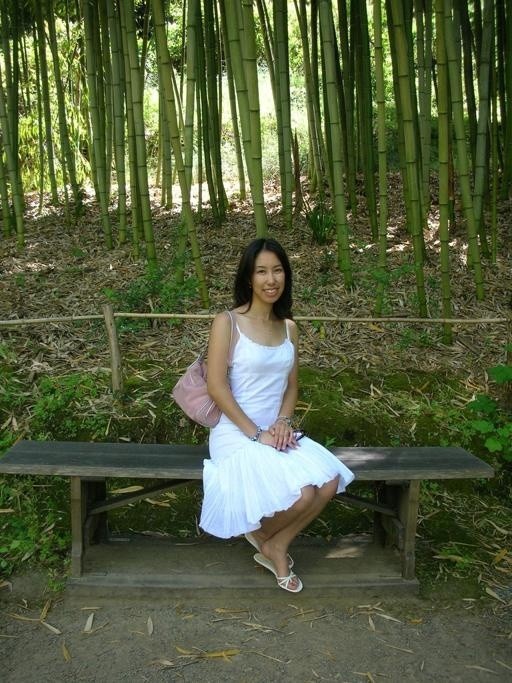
245, 533, 303, 593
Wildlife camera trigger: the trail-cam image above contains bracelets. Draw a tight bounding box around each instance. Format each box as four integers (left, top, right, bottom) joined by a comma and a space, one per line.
276, 416, 292, 427
250, 425, 262, 442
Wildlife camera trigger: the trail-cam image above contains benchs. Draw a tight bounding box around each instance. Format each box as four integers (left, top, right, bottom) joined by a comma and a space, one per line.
2, 439, 500, 597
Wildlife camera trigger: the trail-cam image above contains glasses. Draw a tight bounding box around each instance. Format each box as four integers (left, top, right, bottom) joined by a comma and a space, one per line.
293, 429, 305, 441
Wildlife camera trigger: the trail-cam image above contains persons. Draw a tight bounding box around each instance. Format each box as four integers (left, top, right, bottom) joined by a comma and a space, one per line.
200, 239, 356, 593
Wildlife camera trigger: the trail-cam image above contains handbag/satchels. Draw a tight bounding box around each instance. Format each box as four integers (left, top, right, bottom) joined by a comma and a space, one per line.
172, 353, 233, 427
